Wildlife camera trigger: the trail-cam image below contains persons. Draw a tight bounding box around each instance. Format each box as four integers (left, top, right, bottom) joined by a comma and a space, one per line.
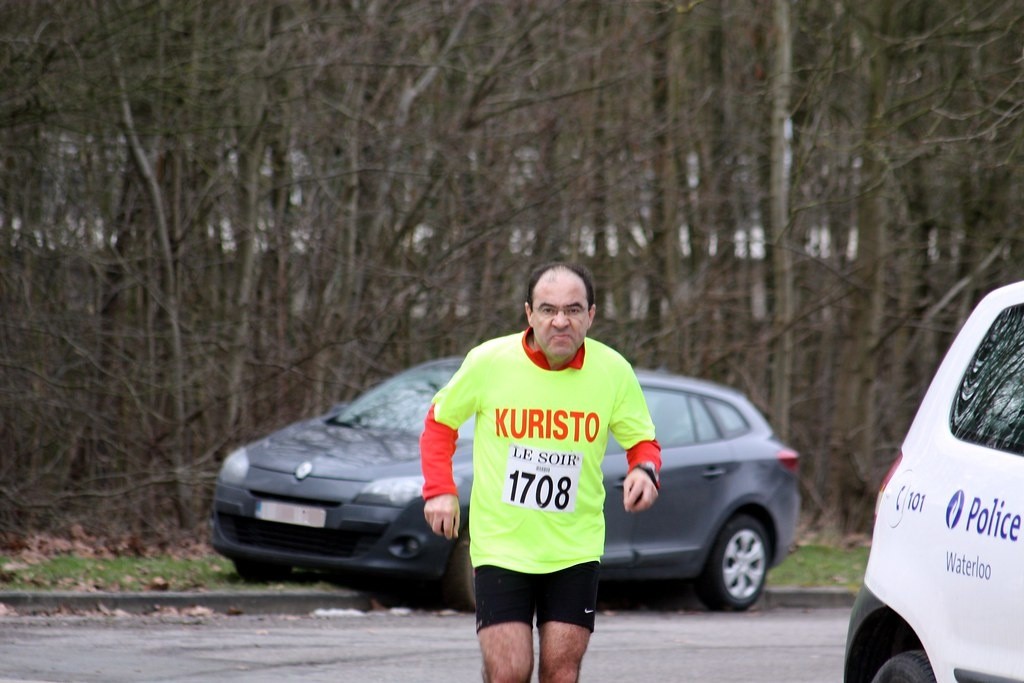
421, 263, 663, 683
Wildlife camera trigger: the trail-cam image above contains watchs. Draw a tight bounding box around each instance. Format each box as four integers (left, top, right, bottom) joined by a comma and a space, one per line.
637, 463, 661, 489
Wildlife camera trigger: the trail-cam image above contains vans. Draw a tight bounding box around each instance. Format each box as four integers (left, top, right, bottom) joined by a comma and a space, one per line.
840, 280, 1024, 683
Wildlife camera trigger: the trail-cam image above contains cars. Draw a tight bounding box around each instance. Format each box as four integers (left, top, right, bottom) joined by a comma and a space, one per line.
208, 352, 804, 615
